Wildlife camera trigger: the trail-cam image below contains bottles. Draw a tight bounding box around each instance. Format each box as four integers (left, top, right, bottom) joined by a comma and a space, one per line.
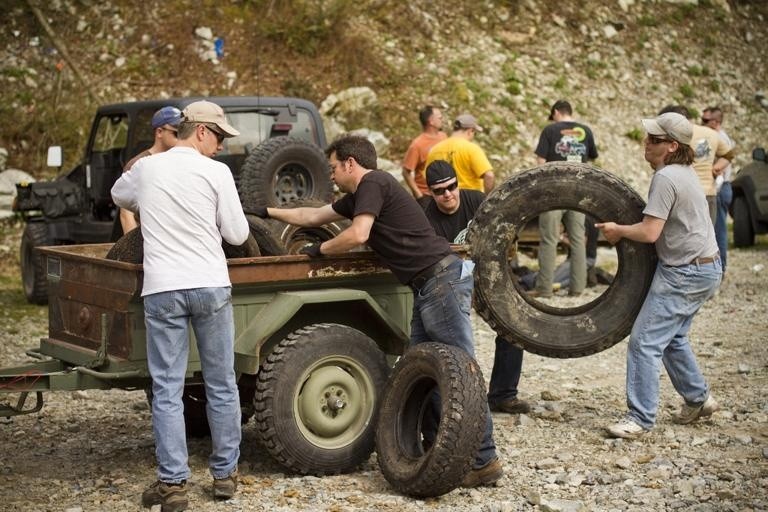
179, 101, 240, 138
641, 112, 693, 146
151, 106, 181, 130
426, 160, 456, 186
549, 100, 570, 120
454, 113, 484, 132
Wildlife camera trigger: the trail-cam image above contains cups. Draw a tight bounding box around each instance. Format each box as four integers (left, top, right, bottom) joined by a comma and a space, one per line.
648, 134, 673, 144
430, 181, 457, 195
161, 127, 178, 138
197, 124, 224, 143
701, 117, 714, 123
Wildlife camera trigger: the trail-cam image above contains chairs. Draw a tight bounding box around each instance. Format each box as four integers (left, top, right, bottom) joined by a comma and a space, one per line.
605, 417, 652, 439
213, 464, 238, 498
461, 457, 503, 489
488, 398, 530, 413
672, 394, 719, 425
141, 479, 188, 512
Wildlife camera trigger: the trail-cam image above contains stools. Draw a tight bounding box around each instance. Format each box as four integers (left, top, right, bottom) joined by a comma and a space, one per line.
409, 254, 459, 291
690, 250, 721, 264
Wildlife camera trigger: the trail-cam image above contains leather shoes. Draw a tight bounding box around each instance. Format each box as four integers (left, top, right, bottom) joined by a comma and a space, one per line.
242, 205, 268, 219
303, 242, 322, 257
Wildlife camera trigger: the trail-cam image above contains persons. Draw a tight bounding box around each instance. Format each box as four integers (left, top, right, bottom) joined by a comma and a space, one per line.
241, 135, 505, 488
425, 161, 533, 413
700, 107, 732, 283
110, 99, 250, 512
403, 106, 449, 212
119, 106, 183, 235
592, 113, 724, 439
659, 104, 734, 228
534, 101, 599, 296
423, 114, 496, 196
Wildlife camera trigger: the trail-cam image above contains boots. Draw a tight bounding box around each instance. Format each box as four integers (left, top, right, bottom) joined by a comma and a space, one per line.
8, 91, 336, 308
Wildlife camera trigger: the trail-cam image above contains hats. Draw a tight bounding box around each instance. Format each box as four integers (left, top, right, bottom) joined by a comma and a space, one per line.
723, 146, 767, 249
